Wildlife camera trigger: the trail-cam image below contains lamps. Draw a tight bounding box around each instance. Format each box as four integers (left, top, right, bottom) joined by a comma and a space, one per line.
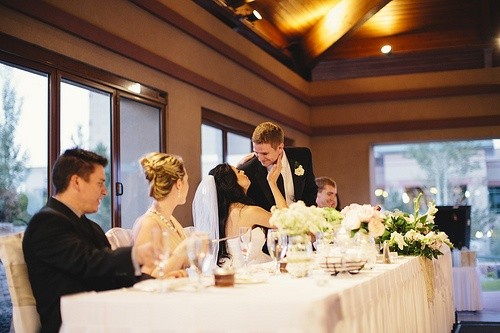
381, 45, 392, 56
248, 10, 263, 22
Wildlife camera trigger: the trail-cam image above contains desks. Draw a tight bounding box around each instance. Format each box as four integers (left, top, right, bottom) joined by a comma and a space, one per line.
60, 244, 481, 333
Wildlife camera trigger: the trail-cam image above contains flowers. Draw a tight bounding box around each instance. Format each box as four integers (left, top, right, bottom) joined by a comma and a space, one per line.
270, 192, 455, 260
294, 161, 304, 176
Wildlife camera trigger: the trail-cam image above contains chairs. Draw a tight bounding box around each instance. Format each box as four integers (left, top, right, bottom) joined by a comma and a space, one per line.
0, 232, 42, 333
105, 228, 133, 249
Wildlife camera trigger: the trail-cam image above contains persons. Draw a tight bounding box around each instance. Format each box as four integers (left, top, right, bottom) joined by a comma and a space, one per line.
192, 152, 290, 272
22, 147, 186, 333
315, 177, 343, 211
238, 122, 315, 254
133, 152, 210, 279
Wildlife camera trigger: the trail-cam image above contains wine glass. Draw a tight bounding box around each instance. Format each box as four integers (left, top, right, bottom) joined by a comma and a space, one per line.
149, 227, 287, 294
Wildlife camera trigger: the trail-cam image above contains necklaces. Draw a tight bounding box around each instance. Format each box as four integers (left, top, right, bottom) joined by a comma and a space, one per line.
151, 209, 177, 232
